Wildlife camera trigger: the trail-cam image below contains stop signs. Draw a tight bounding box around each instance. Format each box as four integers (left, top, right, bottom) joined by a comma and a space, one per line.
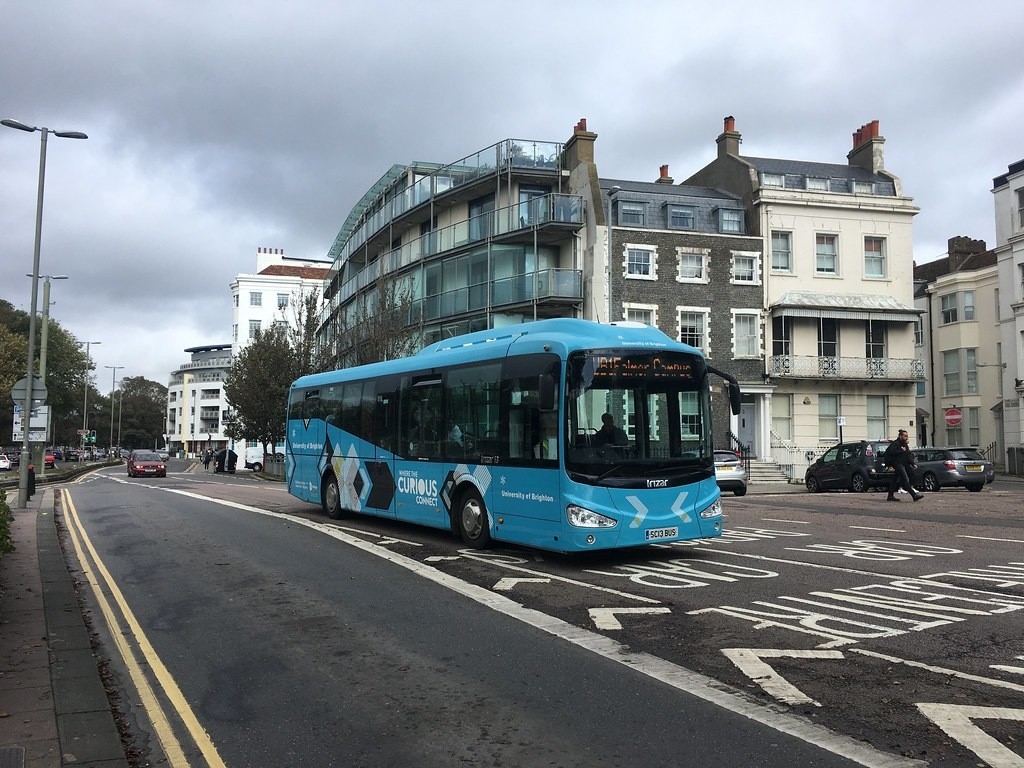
943, 408, 963, 426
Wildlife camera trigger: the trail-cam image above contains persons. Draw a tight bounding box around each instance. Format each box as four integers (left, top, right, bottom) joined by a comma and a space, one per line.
887, 429, 924, 501
449, 419, 463, 447
200, 446, 217, 472
592, 413, 628, 455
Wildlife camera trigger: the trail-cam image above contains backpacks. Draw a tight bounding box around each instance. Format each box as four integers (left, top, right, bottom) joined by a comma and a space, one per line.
884, 441, 897, 466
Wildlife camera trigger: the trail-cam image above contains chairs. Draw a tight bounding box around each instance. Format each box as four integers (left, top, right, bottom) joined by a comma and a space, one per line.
520, 216, 533, 228
575, 427, 599, 449
538, 212, 551, 222
480, 430, 500, 455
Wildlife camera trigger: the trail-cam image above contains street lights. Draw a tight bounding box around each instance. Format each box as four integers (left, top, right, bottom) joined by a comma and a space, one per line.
114, 380, 130, 458
105, 366, 125, 461
1, 118, 90, 508
74, 341, 102, 466
27, 272, 70, 475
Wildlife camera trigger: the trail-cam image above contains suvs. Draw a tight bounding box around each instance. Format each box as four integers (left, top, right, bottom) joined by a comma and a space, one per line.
910, 444, 996, 491
805, 440, 895, 493
713, 449, 748, 496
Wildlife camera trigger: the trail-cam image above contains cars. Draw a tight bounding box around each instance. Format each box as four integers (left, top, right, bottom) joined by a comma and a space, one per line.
156, 449, 170, 461
126, 449, 167, 477
0, 443, 130, 472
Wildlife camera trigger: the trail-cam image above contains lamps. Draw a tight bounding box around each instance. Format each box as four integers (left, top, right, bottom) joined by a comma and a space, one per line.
1015, 378, 1023, 387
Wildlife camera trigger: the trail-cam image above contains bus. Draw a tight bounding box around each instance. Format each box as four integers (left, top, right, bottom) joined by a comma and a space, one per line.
285, 317, 742, 555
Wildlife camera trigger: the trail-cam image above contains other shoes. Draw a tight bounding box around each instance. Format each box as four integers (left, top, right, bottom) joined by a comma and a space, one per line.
887, 497, 900, 501
913, 494, 924, 501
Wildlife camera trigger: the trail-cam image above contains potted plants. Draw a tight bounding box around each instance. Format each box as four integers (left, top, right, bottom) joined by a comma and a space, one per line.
452, 163, 494, 187
552, 197, 577, 221
505, 145, 557, 168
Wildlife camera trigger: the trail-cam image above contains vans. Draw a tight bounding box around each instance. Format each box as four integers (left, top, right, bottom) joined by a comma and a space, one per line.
244, 447, 286, 473
